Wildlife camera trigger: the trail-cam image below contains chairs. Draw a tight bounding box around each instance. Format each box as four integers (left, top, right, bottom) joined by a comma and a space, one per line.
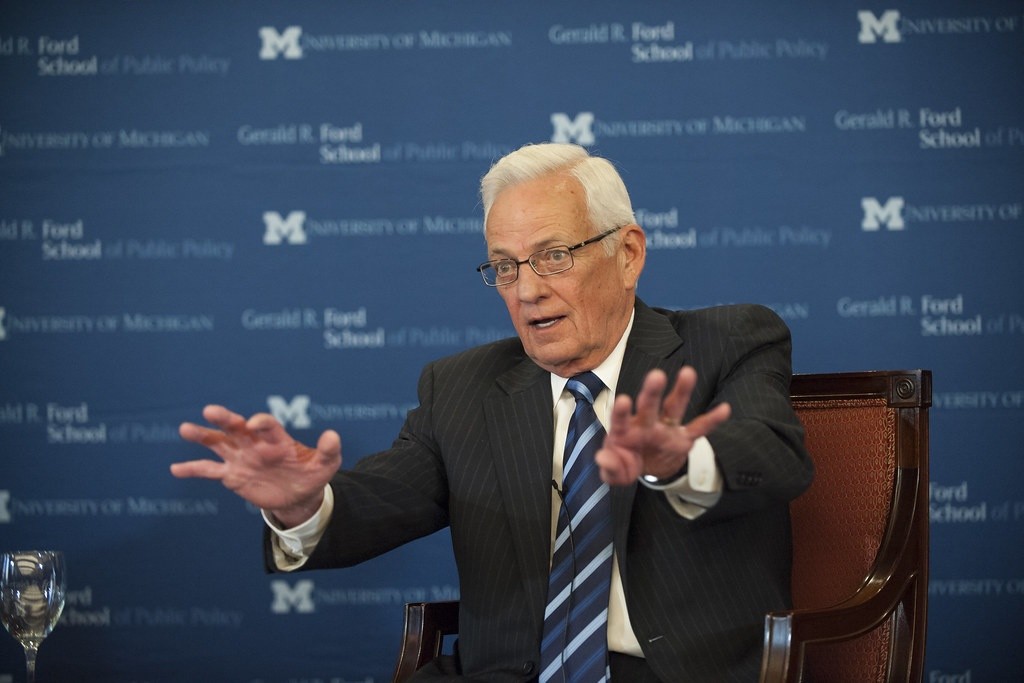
395, 368, 933, 683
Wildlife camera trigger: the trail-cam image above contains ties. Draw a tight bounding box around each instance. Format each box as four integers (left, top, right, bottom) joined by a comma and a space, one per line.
546, 370, 613, 681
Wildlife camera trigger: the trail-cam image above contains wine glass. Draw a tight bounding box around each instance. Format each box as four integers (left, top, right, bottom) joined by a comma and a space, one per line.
0, 549, 66, 683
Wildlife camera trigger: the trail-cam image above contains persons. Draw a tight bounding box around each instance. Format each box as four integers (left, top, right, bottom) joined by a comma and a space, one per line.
169, 141, 815, 683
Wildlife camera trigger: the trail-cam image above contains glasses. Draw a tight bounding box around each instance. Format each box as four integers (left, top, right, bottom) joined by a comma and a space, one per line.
477, 221, 627, 287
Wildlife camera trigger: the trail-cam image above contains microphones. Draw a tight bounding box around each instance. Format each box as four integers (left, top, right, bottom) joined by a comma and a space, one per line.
552, 479, 565, 502
